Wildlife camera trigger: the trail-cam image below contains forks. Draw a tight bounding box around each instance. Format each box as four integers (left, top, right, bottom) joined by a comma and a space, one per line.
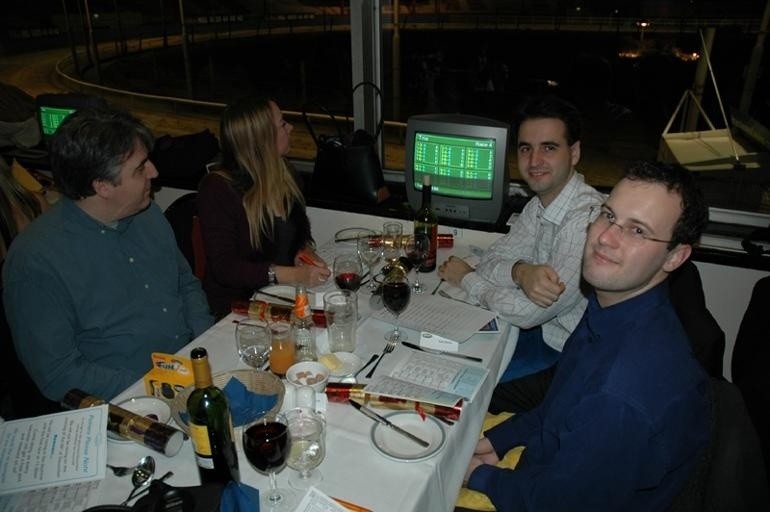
365, 333, 400, 378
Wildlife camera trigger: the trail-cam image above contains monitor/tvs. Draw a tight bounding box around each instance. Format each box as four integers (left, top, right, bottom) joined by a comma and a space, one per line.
35, 93, 107, 150
404, 112, 511, 228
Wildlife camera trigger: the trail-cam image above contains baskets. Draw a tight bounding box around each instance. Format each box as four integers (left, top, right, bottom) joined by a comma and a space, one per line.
177, 367, 284, 451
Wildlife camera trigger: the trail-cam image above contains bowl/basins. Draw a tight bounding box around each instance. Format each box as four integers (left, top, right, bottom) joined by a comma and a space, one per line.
287, 361, 328, 392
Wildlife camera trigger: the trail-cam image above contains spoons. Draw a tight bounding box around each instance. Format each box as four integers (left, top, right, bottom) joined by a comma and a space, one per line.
121, 454, 156, 507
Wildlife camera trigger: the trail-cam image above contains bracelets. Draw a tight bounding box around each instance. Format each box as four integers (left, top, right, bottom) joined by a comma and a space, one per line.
267, 265, 277, 286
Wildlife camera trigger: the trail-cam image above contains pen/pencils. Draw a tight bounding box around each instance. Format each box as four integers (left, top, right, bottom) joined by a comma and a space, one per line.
401, 341, 482, 362
253, 289, 295, 304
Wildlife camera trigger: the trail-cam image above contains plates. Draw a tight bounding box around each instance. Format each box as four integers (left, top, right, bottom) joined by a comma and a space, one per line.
372, 411, 447, 462
254, 284, 320, 310
104, 397, 175, 444
322, 353, 362, 378
335, 226, 375, 247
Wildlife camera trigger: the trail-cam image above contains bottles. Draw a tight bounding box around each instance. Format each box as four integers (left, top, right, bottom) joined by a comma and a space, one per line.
184, 346, 239, 485
290, 285, 318, 363
415, 174, 436, 273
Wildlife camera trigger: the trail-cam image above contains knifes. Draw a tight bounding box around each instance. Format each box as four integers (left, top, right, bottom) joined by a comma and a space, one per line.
402, 339, 484, 364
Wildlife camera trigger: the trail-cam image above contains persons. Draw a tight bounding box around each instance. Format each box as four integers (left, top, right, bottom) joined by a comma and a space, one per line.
435, 96, 610, 387
1, 104, 216, 408
196, 93, 329, 319
465, 156, 720, 511
1, 157, 51, 264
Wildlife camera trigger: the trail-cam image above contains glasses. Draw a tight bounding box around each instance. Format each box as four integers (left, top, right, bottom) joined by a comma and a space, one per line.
588, 203, 678, 247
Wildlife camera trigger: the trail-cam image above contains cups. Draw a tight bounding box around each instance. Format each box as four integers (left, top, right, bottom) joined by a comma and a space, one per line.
323, 290, 359, 350
267, 321, 296, 378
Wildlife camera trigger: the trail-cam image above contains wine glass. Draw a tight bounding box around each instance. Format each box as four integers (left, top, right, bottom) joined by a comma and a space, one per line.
333, 254, 362, 322
283, 405, 329, 489
357, 220, 431, 296
380, 277, 413, 344
236, 317, 272, 394
241, 411, 297, 511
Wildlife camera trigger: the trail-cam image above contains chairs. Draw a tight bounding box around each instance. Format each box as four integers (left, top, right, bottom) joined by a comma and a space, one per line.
0, 272, 74, 426
162, 186, 220, 322
657, 245, 725, 373
670, 372, 761, 510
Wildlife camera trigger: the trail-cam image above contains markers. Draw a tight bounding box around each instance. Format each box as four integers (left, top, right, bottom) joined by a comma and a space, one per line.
299, 255, 315, 266
